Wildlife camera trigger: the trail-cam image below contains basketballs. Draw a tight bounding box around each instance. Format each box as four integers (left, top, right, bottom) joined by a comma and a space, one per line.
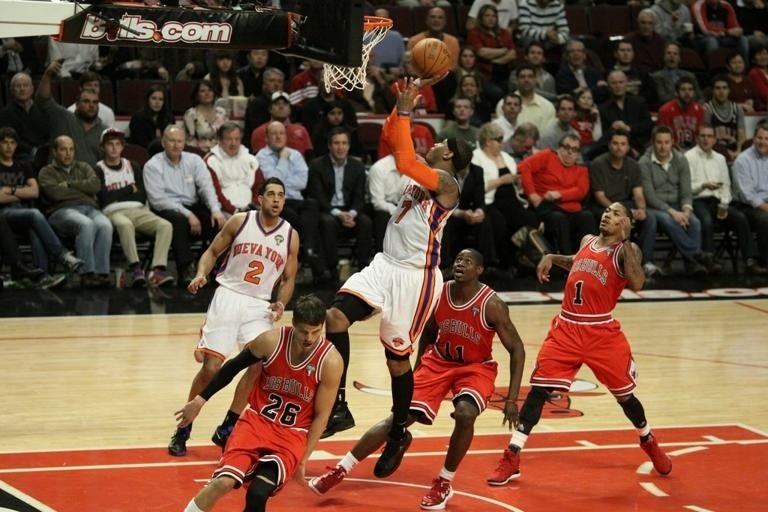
411, 37, 452, 81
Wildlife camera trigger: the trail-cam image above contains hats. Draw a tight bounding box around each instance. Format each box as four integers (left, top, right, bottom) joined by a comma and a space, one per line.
100, 128, 125, 148
272, 90, 291, 104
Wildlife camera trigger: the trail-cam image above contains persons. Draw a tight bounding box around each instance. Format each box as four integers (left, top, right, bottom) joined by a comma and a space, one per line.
1, 1, 767, 296
307, 246, 525, 511
168, 176, 302, 457
485, 201, 672, 485
174, 295, 344, 512
318, 70, 472, 477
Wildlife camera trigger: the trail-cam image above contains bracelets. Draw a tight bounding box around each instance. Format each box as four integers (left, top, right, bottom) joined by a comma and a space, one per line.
277, 301, 285, 310
506, 398, 516, 404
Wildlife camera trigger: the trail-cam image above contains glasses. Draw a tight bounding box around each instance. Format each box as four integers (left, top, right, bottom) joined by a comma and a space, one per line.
561, 144, 578, 152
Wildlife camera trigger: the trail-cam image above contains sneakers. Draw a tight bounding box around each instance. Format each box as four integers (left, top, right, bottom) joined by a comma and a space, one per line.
309, 466, 346, 496
131, 268, 174, 287
178, 274, 193, 287
687, 260, 768, 277
419, 476, 452, 511
168, 423, 192, 456
487, 448, 521, 486
212, 427, 228, 445
374, 429, 412, 478
639, 431, 671, 475
644, 262, 662, 278
319, 401, 355, 439
11, 254, 112, 290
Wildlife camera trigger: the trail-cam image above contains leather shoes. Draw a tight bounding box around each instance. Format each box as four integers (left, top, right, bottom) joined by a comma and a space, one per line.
314, 269, 331, 283
301, 247, 319, 259
